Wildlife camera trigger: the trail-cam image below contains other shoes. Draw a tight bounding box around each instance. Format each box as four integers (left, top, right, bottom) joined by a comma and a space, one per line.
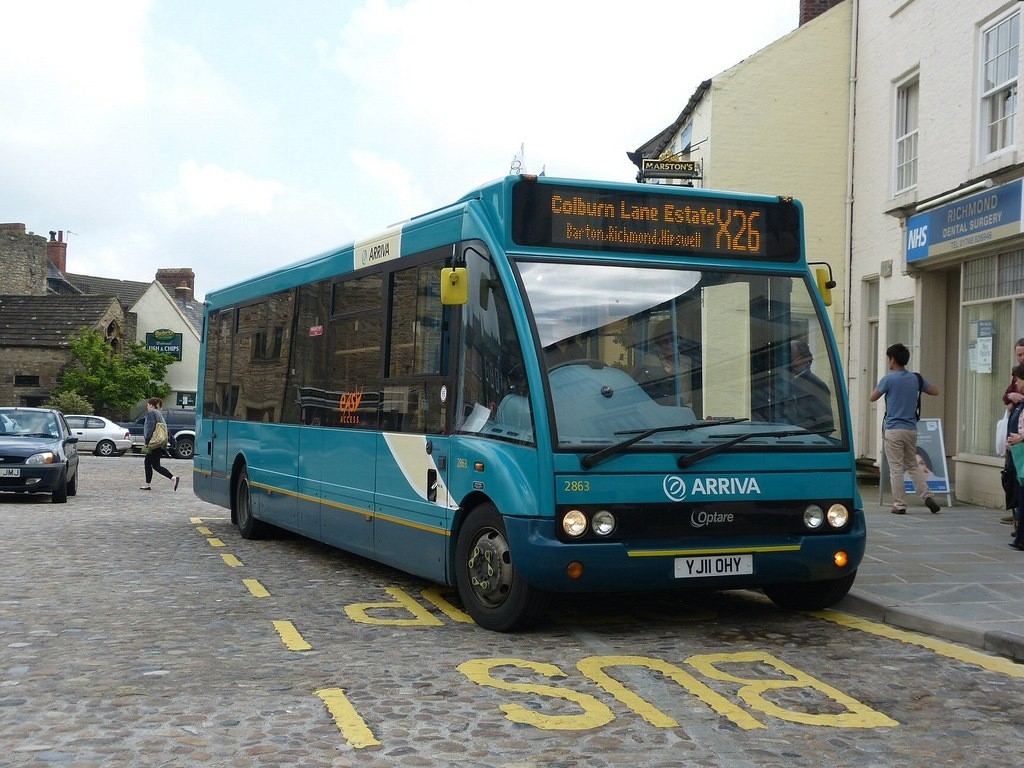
1001, 514, 1024, 552
926, 498, 940, 514
173, 476, 180, 491
138, 486, 151, 491
891, 505, 907, 515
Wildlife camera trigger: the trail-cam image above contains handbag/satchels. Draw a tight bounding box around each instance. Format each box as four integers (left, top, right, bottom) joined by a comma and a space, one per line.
994, 408, 1011, 457
140, 445, 153, 455
149, 422, 167, 450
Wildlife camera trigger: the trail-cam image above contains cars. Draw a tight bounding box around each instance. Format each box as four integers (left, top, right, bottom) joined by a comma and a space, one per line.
0, 406, 80, 503
49, 414, 131, 457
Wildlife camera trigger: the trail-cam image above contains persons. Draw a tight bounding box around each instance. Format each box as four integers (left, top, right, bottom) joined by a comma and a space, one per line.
903, 446, 934, 479
138, 396, 181, 492
995, 337, 1023, 534
867, 342, 942, 516
1005, 364, 1024, 551
642, 317, 694, 409
786, 337, 837, 439
528, 293, 585, 371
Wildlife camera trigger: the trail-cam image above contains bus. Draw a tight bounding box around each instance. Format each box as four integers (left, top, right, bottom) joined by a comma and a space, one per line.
193, 174, 868, 630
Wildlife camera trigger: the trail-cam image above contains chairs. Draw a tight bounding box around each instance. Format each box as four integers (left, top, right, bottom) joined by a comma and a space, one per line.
26, 419, 53, 440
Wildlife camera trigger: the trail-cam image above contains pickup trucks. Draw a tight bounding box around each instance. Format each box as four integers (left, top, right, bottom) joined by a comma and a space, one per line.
116, 407, 197, 459
